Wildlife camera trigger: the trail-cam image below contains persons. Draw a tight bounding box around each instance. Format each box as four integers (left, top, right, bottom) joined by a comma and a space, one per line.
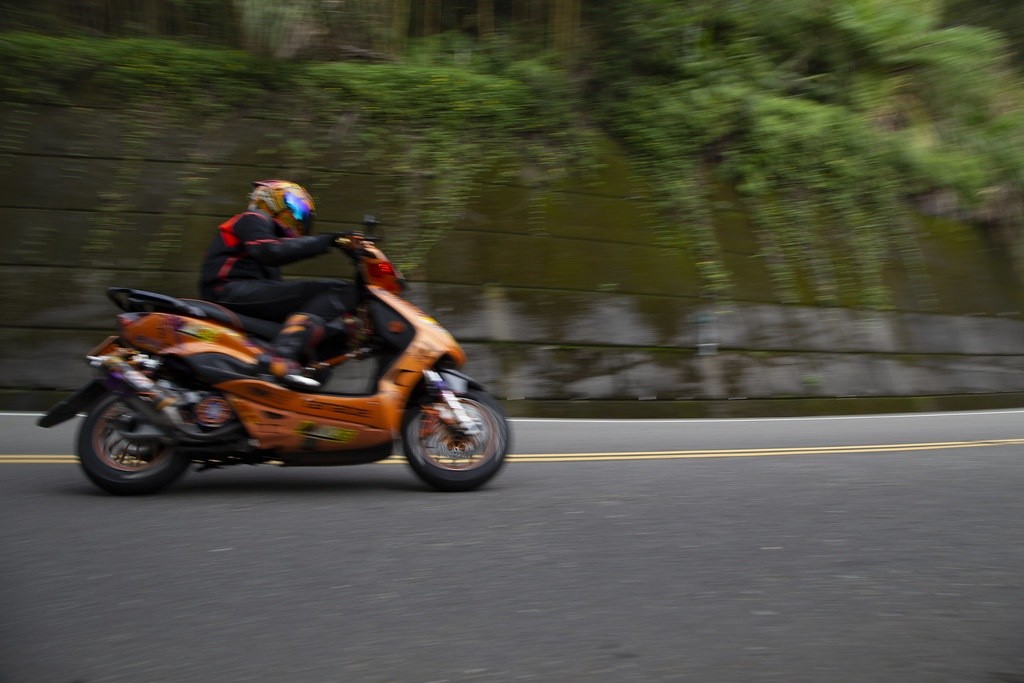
201, 180, 375, 386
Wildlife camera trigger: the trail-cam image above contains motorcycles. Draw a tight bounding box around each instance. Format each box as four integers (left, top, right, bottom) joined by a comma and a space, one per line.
34, 230, 513, 496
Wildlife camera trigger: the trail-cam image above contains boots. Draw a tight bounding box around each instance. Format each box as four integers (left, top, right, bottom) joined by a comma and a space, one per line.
257, 312, 327, 388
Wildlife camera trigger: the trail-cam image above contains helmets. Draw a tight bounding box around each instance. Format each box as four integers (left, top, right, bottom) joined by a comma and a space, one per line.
247, 178, 316, 238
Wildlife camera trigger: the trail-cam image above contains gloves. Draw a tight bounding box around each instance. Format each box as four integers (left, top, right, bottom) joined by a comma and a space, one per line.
334, 231, 361, 250
